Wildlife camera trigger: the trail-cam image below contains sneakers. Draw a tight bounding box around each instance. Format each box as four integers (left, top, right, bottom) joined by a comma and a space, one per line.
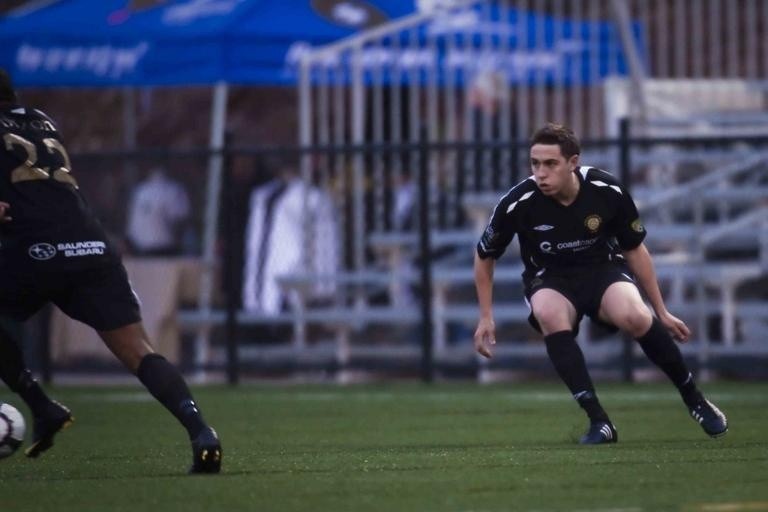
24, 398, 74, 459
689, 390, 728, 438
188, 425, 221, 474
580, 419, 619, 444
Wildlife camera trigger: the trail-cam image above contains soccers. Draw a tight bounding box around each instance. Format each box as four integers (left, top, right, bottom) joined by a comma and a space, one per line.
0, 402, 26, 460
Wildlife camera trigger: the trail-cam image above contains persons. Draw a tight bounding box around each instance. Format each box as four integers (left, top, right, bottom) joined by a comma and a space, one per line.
473, 120, 729, 445
0, 72, 225, 474
127, 159, 191, 255
332, 152, 468, 272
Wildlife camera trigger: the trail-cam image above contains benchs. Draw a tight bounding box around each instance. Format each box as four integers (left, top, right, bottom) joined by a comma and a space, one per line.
196, 123, 768, 374
